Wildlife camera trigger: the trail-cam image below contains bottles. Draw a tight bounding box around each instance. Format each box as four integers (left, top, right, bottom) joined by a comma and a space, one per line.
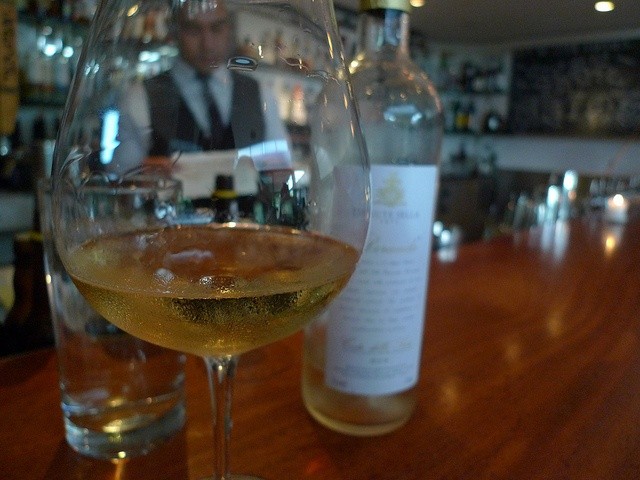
300, 2, 445, 438
43, 0, 191, 460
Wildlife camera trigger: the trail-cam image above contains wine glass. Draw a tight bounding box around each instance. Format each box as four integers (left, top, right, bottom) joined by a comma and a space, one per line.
48, 0, 373, 480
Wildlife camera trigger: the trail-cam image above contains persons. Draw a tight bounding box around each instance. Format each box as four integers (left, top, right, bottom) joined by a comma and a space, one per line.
111, 0, 294, 188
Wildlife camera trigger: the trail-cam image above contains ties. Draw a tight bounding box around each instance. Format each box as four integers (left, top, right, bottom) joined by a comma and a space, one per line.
194, 71, 229, 148
